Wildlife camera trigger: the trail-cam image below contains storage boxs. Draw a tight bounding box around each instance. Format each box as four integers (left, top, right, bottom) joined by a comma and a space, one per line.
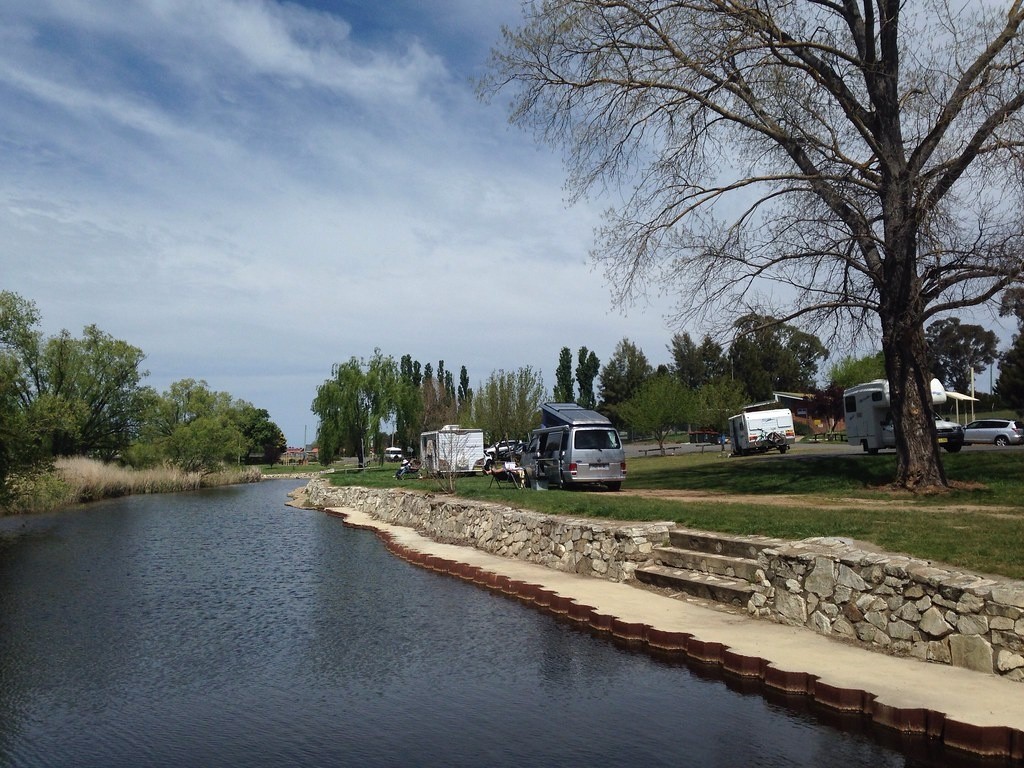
530, 477, 550, 491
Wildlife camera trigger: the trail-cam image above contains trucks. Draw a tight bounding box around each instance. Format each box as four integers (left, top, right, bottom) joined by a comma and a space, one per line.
844, 378, 964, 455
728, 409, 795, 456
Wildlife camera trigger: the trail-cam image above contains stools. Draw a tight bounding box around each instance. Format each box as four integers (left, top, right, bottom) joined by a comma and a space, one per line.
815, 432, 827, 441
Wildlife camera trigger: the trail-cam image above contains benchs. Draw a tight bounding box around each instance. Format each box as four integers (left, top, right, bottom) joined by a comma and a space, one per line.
638, 445, 681, 455
696, 443, 726, 454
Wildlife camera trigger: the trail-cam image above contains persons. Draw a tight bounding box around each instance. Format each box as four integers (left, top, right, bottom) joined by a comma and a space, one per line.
397, 456, 417, 480
483, 453, 522, 475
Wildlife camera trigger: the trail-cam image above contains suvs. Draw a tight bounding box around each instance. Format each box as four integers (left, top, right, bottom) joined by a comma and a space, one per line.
485, 439, 522, 459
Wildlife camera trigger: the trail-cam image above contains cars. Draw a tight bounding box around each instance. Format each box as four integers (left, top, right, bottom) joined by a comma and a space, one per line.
498, 442, 529, 462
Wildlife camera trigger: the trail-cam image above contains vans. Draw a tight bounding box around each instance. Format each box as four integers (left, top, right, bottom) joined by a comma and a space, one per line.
385, 447, 403, 462
519, 403, 628, 491
962, 418, 1024, 446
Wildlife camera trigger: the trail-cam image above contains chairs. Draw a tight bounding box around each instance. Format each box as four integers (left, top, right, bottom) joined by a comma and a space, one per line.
486, 464, 525, 489
